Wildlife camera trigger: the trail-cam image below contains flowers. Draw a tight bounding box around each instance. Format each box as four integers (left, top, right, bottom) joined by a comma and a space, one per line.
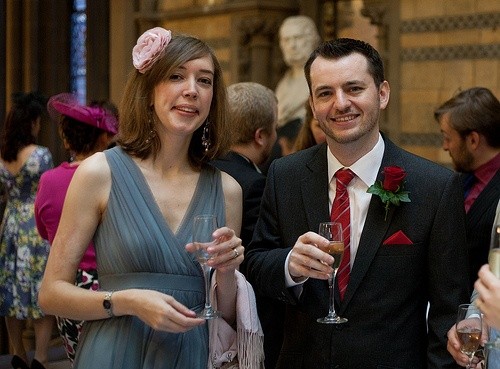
132, 27, 171, 74
366, 166, 411, 220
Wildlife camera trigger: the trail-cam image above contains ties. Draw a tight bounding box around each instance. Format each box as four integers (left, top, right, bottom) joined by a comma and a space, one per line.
330, 169, 356, 290
464, 174, 477, 200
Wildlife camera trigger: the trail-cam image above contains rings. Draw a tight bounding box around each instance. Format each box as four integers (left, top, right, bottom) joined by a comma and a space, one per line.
233, 248, 240, 257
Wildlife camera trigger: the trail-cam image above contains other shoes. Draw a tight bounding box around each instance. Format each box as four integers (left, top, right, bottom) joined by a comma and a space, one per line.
11, 355, 45, 369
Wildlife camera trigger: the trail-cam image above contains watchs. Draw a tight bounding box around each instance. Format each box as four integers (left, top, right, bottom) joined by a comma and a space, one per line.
102, 290, 116, 319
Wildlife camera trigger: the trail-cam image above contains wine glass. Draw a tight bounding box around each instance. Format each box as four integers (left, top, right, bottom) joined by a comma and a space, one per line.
192, 215, 223, 319
455, 303, 481, 369
316, 223, 348, 324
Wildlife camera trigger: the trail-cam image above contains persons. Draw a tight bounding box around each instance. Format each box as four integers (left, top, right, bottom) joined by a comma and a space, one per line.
38, 26, 243, 369
434, 87, 500, 293
208, 80, 278, 245
35, 101, 119, 367
0, 92, 61, 369
244, 37, 472, 369
274, 14, 322, 128
446, 263, 500, 369
290, 97, 326, 152
